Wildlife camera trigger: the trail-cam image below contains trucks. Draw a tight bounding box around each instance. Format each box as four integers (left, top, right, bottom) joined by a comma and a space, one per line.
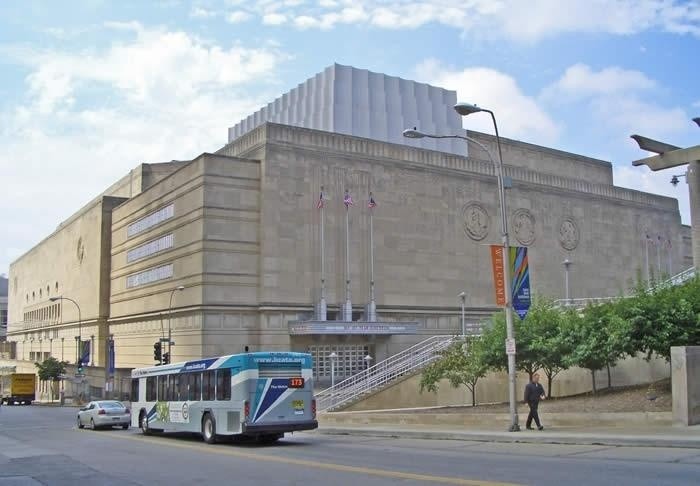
0, 373, 36, 405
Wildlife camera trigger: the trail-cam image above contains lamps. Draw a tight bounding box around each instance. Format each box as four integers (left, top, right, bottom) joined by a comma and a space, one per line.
670, 174, 686, 187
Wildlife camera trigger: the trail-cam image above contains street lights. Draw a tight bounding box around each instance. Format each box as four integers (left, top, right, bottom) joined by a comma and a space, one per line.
169, 285, 184, 359
49, 296, 82, 366
401, 103, 520, 432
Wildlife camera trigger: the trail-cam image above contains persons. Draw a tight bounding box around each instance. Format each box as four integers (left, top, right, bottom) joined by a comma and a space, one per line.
524, 373, 546, 431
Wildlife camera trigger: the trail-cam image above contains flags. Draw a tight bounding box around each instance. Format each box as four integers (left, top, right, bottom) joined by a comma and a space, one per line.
315, 190, 324, 209
368, 196, 378, 209
343, 193, 355, 208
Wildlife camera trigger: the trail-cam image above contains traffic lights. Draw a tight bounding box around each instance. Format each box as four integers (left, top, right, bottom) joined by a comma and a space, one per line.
77, 359, 81, 371
153, 342, 160, 362
162, 352, 169, 365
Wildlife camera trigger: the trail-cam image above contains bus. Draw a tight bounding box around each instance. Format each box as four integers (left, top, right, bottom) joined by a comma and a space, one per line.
130, 352, 319, 444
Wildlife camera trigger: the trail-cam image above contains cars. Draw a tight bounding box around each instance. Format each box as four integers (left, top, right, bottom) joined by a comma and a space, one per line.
75, 398, 131, 431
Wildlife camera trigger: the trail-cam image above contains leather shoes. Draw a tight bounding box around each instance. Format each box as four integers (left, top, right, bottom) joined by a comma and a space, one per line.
538, 425, 543, 430
526, 426, 534, 430
